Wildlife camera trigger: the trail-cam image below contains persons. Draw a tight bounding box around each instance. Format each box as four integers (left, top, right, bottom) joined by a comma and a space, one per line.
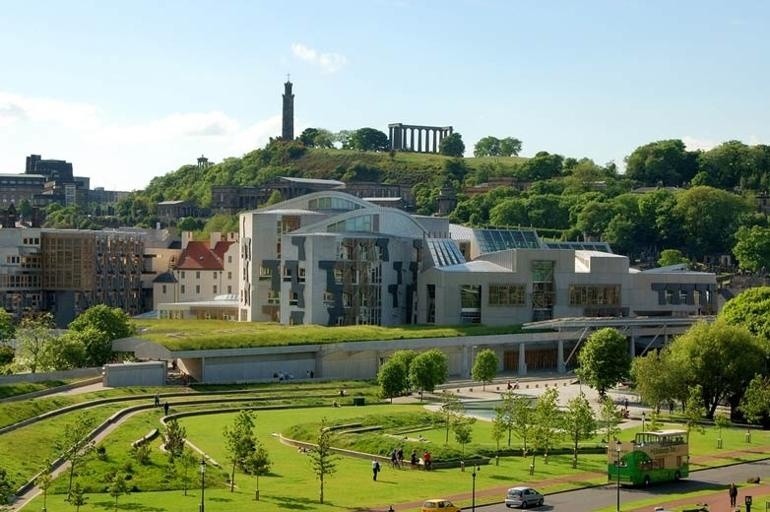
154, 391, 160, 407
164, 401, 169, 416
729, 482, 737, 507
273, 370, 294, 383
307, 367, 314, 379
641, 411, 645, 422
620, 398, 629, 418
372, 457, 378, 481
391, 446, 433, 470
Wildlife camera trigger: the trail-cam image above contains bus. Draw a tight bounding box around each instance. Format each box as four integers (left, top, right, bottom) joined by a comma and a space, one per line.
607, 429, 689, 489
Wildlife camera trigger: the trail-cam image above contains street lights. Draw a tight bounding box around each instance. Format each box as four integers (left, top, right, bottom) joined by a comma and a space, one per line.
470, 344, 479, 368
639, 411, 647, 442
471, 463, 481, 512
745, 495, 753, 512
199, 459, 208, 511
614, 439, 623, 512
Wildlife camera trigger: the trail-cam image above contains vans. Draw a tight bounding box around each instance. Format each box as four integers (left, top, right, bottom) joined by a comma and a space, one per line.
505, 487, 545, 509
421, 498, 461, 512
